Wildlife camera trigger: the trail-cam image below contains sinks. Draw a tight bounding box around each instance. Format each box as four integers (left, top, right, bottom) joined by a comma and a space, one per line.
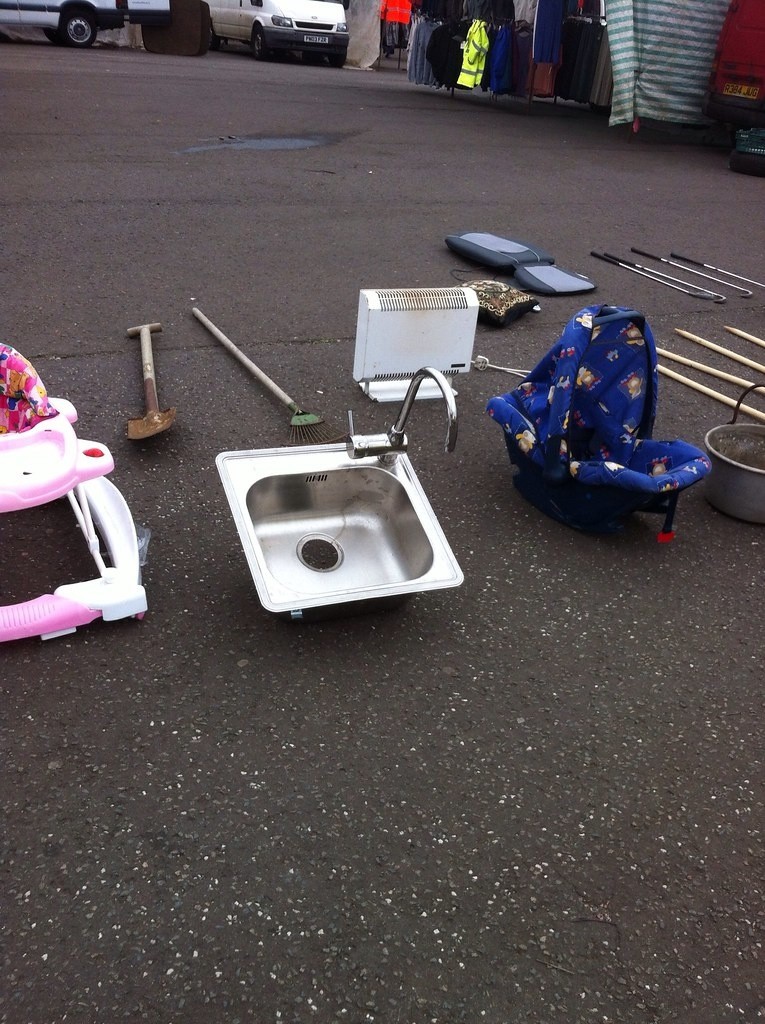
212, 433, 467, 617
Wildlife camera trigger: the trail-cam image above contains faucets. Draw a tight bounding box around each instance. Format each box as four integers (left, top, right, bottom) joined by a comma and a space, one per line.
389, 365, 456, 456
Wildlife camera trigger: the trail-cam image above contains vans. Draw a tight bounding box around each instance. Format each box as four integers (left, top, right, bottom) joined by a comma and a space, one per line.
201, 0, 349, 67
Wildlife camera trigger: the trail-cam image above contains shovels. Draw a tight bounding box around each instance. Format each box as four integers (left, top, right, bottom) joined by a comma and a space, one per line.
126, 321, 177, 439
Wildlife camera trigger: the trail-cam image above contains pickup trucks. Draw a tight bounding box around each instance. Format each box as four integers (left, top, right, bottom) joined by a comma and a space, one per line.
0, 0, 172, 48
